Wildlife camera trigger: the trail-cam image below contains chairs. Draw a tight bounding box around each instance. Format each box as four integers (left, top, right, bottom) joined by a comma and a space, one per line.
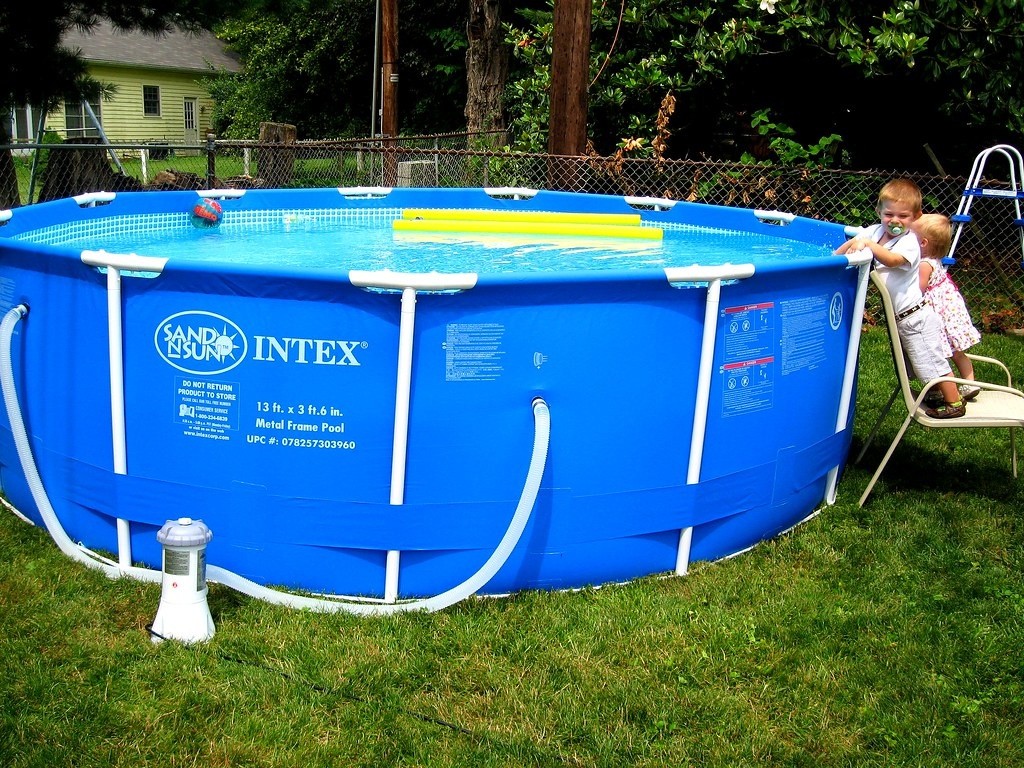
853, 270, 1024, 511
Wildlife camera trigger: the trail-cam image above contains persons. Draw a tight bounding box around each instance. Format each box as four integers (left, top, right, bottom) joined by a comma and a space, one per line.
910, 214, 981, 400
834, 178, 967, 419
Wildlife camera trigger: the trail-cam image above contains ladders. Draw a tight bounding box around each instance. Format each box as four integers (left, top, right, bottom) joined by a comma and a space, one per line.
939, 142, 1024, 278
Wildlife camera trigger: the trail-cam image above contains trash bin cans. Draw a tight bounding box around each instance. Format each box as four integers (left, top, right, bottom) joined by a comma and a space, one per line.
148, 141, 169, 160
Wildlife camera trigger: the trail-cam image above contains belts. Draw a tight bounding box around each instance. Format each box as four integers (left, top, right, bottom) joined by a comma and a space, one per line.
895, 299, 927, 320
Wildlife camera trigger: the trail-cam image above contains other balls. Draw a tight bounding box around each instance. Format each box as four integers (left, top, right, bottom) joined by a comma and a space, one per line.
189, 197, 223, 230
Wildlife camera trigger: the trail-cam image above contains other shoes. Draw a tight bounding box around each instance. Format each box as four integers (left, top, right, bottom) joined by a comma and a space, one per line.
926, 403, 966, 419
926, 395, 967, 409
959, 384, 980, 400
